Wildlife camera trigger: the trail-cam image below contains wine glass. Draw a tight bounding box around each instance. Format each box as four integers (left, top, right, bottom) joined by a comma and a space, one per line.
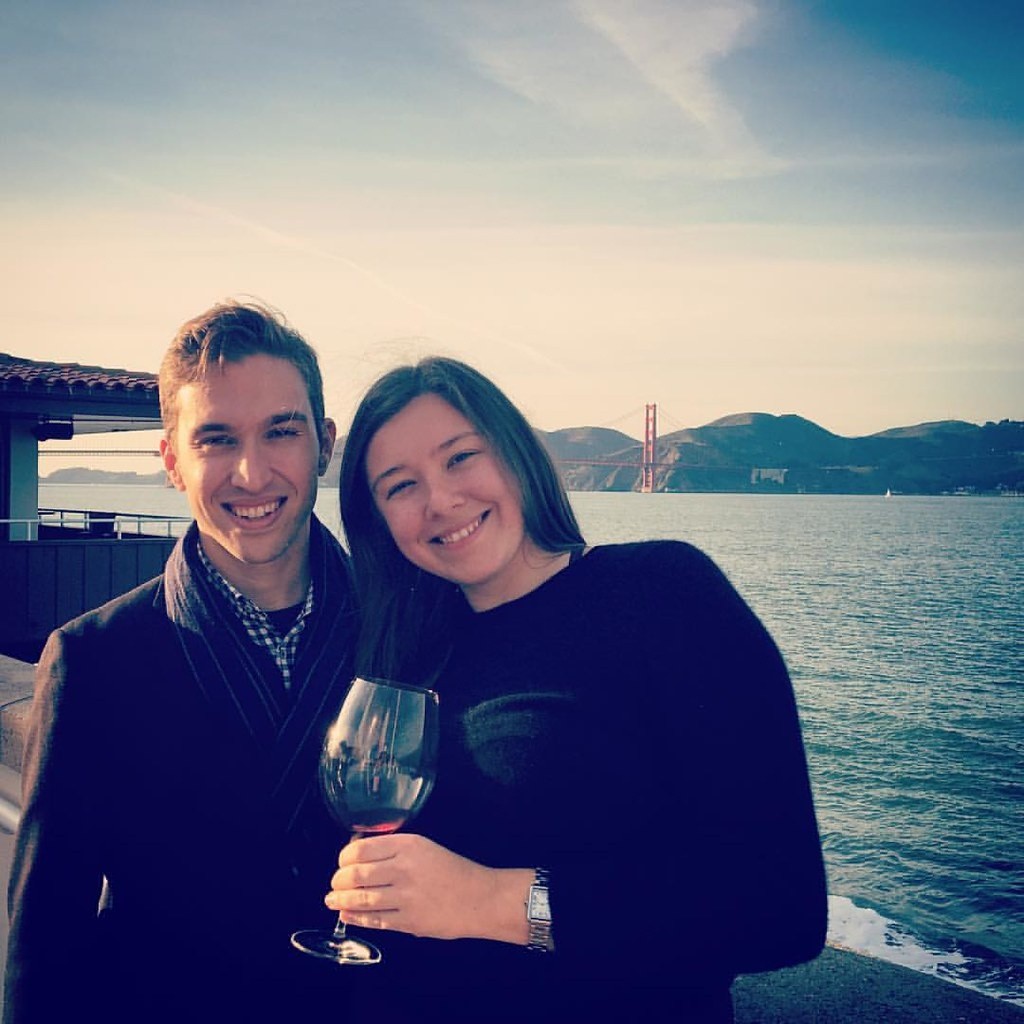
290, 674, 440, 966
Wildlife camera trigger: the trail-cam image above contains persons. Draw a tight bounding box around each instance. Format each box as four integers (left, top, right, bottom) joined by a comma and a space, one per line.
0, 304, 350, 1024
324, 357, 830, 1024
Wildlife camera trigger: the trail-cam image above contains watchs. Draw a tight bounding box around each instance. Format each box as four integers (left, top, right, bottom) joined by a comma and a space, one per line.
523, 864, 552, 954
